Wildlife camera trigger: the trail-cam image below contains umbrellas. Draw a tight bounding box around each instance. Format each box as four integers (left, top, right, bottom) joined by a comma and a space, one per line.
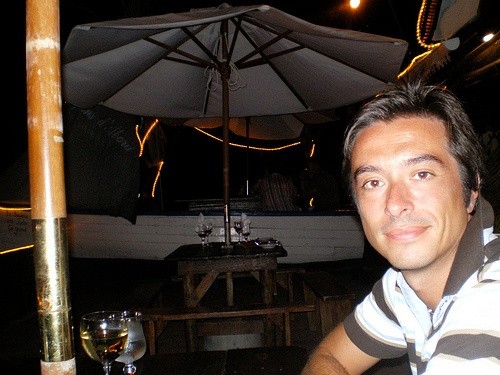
67, 5, 407, 248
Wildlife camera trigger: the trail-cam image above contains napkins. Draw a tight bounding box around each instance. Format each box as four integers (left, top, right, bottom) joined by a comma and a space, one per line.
194, 212, 205, 233
242, 213, 250, 233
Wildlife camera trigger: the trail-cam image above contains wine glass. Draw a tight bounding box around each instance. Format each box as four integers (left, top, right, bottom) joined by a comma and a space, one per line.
196, 221, 213, 249
233, 220, 249, 246
79, 311, 131, 375
114, 311, 147, 375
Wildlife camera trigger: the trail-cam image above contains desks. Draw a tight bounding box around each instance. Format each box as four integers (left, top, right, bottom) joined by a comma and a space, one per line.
76, 344, 310, 375
162, 239, 288, 352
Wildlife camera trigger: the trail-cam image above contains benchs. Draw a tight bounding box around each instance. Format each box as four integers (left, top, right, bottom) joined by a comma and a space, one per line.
124, 268, 348, 355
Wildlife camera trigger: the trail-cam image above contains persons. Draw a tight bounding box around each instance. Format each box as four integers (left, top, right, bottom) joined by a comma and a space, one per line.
250, 80, 499, 375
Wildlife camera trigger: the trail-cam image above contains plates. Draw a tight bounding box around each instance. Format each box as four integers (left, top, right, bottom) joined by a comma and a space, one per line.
259, 243, 278, 248
